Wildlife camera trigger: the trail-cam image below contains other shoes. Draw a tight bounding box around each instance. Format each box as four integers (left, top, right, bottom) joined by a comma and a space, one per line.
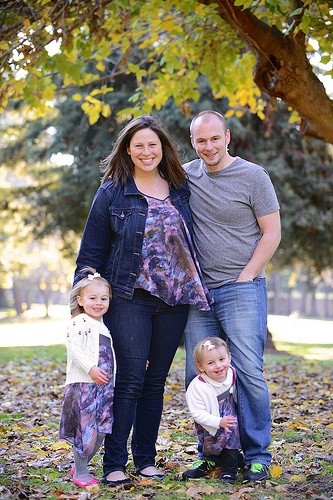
104, 476, 131, 486
220, 472, 237, 484
136, 470, 168, 481
72, 474, 98, 488
70, 464, 99, 484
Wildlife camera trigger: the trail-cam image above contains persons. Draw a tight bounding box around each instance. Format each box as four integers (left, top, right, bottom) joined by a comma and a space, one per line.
57, 265, 115, 488
74, 115, 215, 484
185, 337, 238, 483
182, 110, 282, 483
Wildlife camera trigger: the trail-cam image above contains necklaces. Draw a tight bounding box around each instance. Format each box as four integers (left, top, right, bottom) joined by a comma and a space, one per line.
133, 174, 163, 191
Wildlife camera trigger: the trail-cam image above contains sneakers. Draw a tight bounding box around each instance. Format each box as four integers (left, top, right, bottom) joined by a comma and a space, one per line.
238, 451, 248, 470
182, 460, 221, 479
241, 463, 269, 485
